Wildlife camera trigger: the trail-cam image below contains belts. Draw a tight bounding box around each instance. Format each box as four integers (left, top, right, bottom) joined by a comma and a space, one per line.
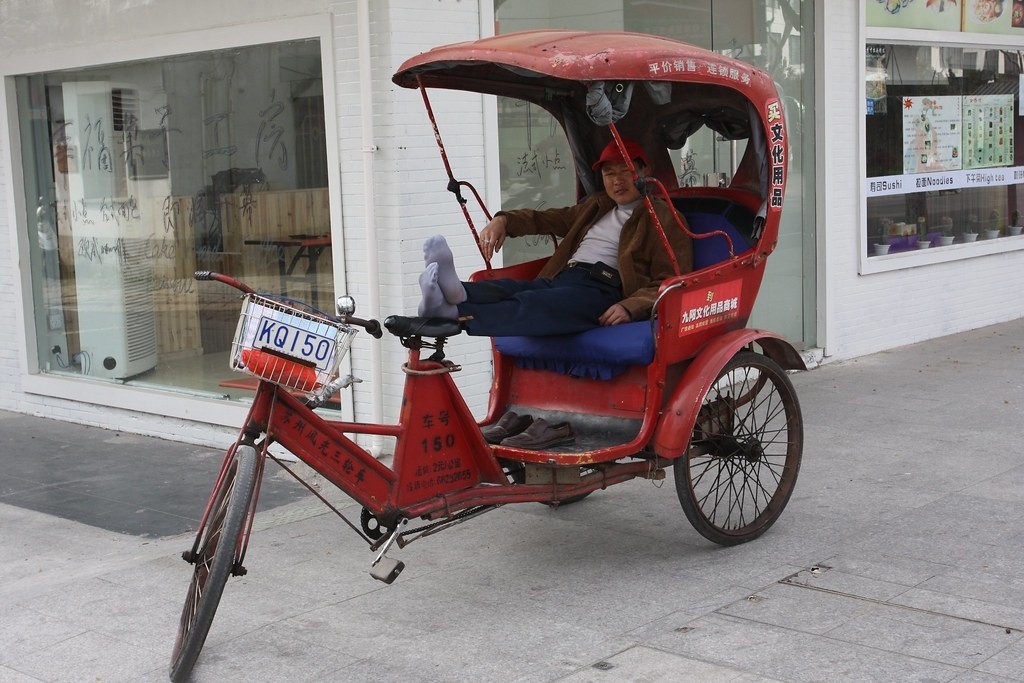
567, 262, 594, 272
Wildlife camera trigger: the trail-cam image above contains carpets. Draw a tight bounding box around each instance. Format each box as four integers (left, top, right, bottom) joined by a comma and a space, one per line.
217, 377, 340, 408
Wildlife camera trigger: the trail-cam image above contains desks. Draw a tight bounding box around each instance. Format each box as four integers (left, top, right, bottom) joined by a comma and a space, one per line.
245, 233, 332, 321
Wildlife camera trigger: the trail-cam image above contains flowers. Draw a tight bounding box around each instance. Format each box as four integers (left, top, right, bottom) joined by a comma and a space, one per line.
877, 217, 894, 245
965, 215, 977, 233
941, 216, 954, 236
986, 209, 1000, 230
917, 216, 928, 240
1009, 209, 1023, 227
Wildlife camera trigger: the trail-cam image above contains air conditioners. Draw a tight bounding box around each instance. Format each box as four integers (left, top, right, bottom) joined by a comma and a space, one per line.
61, 80, 159, 378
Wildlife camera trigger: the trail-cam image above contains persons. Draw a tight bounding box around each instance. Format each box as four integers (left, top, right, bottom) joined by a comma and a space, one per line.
418, 141, 693, 336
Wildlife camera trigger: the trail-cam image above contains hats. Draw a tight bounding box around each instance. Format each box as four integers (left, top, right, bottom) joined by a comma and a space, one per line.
592, 137, 648, 171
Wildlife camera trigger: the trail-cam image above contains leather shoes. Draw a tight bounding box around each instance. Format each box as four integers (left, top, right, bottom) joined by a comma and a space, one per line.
500, 418, 575, 449
482, 411, 534, 443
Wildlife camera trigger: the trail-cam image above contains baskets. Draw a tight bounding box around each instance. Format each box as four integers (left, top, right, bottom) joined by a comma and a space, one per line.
229, 293, 359, 396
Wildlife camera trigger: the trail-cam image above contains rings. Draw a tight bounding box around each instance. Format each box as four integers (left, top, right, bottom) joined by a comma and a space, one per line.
484, 239, 490, 243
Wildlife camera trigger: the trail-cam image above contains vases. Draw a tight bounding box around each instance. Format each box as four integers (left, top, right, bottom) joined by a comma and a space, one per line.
874, 243, 891, 255
984, 229, 1000, 239
1008, 226, 1023, 235
917, 240, 932, 249
963, 232, 980, 244
939, 236, 956, 245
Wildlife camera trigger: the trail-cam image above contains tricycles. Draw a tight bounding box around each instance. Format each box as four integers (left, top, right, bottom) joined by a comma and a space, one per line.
163, 30, 810, 683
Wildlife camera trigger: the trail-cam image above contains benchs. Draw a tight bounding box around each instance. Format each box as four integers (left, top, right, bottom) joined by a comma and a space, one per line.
494, 196, 760, 381
